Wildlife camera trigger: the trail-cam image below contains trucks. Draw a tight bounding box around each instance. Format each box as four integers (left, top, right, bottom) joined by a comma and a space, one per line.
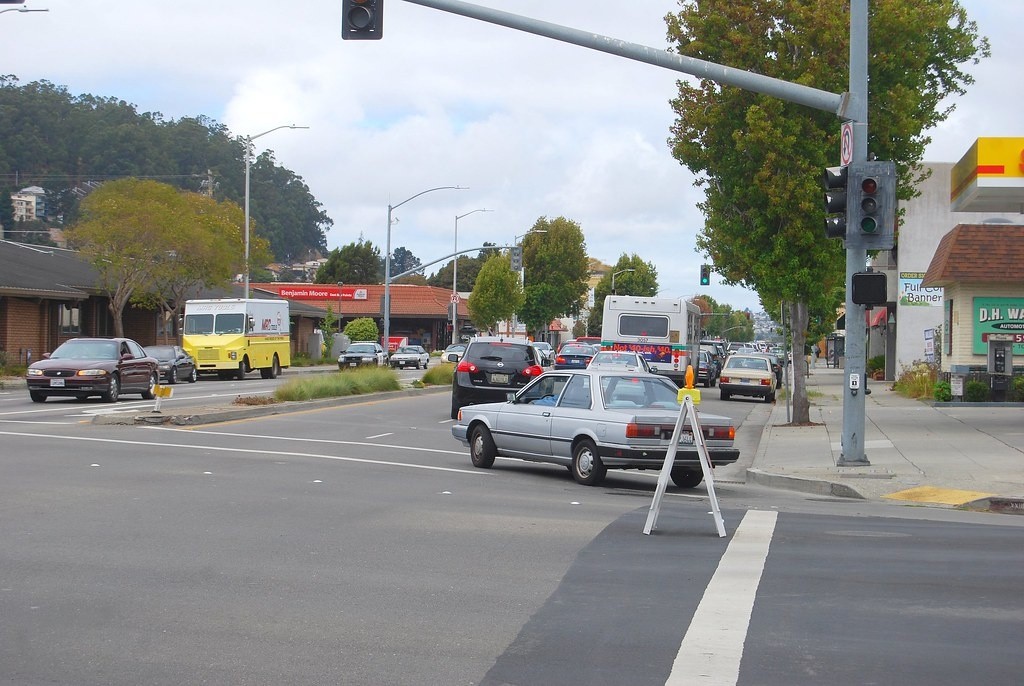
601, 295, 707, 388
177, 299, 291, 382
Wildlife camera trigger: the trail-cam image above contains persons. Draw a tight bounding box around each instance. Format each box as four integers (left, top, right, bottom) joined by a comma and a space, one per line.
531, 376, 584, 406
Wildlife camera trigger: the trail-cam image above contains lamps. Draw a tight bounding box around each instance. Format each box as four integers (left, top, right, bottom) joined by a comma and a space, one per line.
888, 312, 897, 338
878, 318, 885, 337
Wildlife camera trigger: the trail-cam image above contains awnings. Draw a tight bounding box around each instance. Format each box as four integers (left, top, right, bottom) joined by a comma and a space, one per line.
871, 308, 886, 326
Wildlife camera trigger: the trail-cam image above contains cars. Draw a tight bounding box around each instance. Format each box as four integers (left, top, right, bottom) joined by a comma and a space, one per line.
389, 345, 430, 370
26, 336, 161, 403
337, 340, 389, 369
143, 345, 197, 384
458, 333, 556, 368
697, 340, 792, 388
582, 349, 658, 405
451, 370, 741, 489
450, 342, 551, 420
556, 336, 601, 352
554, 345, 595, 371
440, 343, 469, 363
719, 355, 779, 404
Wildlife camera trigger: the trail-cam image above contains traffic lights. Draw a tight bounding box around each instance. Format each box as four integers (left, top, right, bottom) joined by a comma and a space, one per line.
852, 272, 888, 305
700, 265, 710, 285
341, 0, 384, 41
845, 160, 895, 249
821, 164, 849, 238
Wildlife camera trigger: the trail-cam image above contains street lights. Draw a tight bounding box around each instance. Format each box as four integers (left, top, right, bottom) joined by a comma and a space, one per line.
612, 269, 635, 295
452, 208, 495, 344
337, 281, 343, 334
244, 123, 310, 299
511, 229, 548, 339
383, 183, 471, 353
